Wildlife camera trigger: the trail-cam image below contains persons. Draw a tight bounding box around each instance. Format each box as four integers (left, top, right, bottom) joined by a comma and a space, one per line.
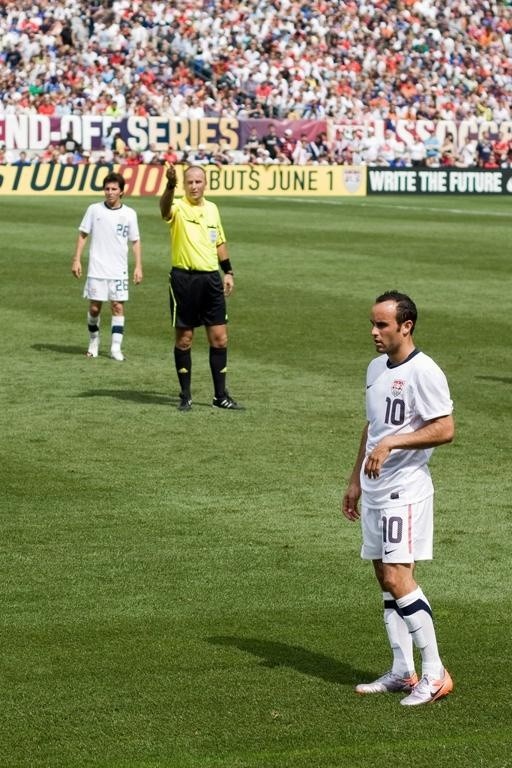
1, 0, 512, 168
69, 172, 144, 362
160, 164, 247, 411
340, 288, 457, 708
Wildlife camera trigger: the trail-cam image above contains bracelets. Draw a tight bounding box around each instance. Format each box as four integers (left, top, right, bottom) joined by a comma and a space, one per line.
219, 258, 235, 275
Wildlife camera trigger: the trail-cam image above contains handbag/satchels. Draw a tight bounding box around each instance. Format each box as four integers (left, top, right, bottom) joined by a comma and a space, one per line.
219, 258, 235, 275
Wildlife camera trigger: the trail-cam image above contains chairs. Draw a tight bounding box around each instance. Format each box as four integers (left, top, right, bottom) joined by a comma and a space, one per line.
178, 394, 194, 410
109, 352, 126, 361
213, 395, 243, 408
355, 668, 417, 694
88, 338, 100, 359
402, 670, 452, 707
